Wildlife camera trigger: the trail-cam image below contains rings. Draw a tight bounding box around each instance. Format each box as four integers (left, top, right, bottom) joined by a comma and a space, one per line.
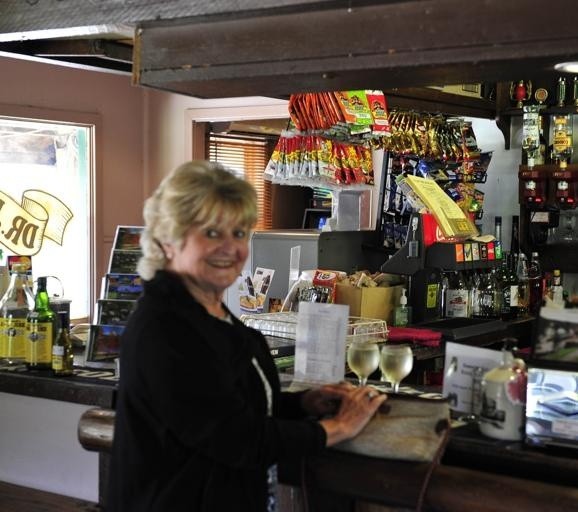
367, 392, 374, 399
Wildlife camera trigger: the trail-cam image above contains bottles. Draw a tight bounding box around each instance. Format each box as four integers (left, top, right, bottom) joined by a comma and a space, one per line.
440, 251, 563, 318
52, 311, 74, 377
28, 273, 56, 369
510, 214, 519, 253
0, 263, 35, 373
492, 214, 502, 242
523, 113, 540, 169
555, 75, 578, 108
476, 336, 528, 442
553, 116, 573, 168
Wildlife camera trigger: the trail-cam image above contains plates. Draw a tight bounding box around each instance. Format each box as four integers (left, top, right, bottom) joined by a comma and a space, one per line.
534, 88, 548, 103
240, 306, 256, 312
257, 306, 263, 309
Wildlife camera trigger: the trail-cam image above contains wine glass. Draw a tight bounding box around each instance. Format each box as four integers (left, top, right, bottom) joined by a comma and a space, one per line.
380, 346, 412, 395
348, 342, 380, 388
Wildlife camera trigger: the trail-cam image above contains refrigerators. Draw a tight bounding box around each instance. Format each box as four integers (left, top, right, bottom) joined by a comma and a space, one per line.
250, 230, 383, 315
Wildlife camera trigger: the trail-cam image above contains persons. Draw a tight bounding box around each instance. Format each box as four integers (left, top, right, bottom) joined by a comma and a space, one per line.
109, 159, 388, 512
